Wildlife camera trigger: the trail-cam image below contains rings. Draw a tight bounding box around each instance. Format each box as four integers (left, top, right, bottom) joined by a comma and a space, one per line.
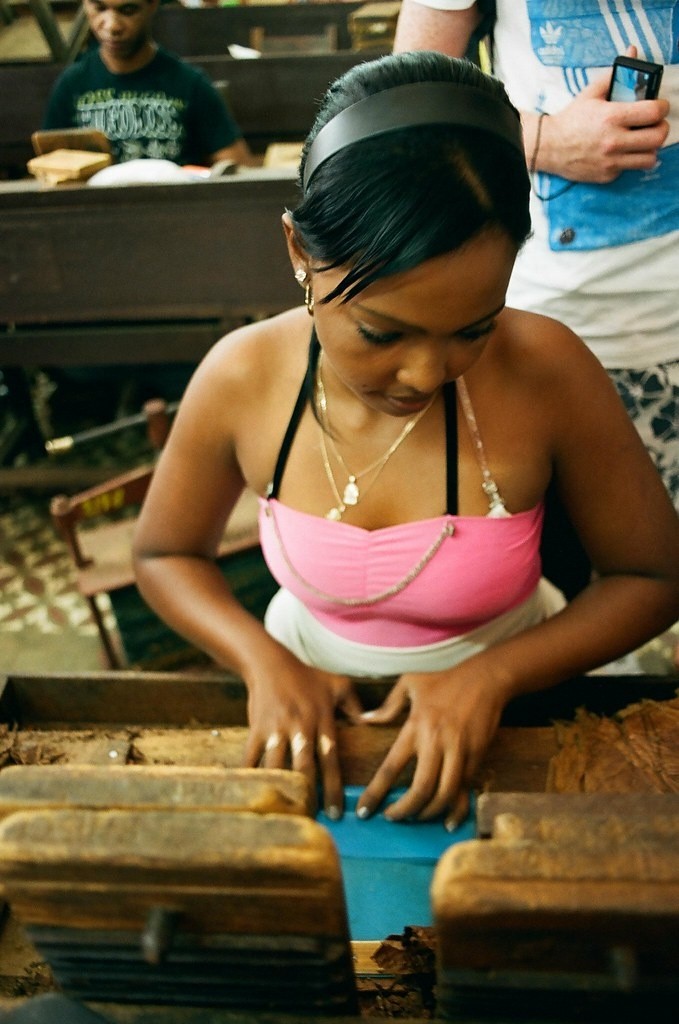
262, 736, 285, 752
316, 733, 337, 757
289, 732, 315, 754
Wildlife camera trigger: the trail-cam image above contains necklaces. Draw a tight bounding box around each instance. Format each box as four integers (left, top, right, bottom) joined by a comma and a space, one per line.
303, 333, 444, 521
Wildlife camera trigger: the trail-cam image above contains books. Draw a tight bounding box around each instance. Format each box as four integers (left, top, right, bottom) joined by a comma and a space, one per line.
26, 129, 113, 182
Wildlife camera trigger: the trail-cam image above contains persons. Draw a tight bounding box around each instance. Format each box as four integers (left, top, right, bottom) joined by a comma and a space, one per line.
130, 49, 679, 834
386, 0, 679, 677
36, 1, 250, 170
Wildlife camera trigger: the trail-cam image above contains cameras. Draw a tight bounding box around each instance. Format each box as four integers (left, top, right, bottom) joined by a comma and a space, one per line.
606, 55, 664, 131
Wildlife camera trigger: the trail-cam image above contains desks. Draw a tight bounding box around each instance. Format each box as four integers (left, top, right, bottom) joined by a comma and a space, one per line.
0, 55, 386, 499
0, 672, 679, 1024
